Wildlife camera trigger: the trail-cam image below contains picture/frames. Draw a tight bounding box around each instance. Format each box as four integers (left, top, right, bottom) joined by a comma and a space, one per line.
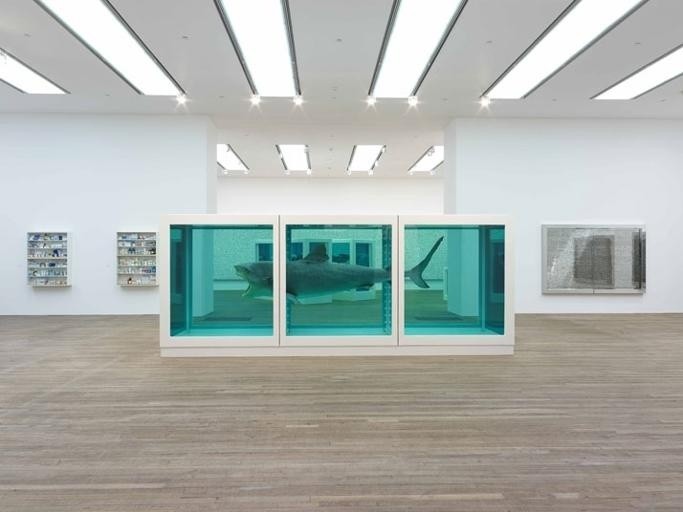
540, 225, 646, 295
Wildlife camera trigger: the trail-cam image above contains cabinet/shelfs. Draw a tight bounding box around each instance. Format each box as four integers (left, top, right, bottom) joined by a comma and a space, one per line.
116, 229, 160, 289
26, 231, 73, 288
